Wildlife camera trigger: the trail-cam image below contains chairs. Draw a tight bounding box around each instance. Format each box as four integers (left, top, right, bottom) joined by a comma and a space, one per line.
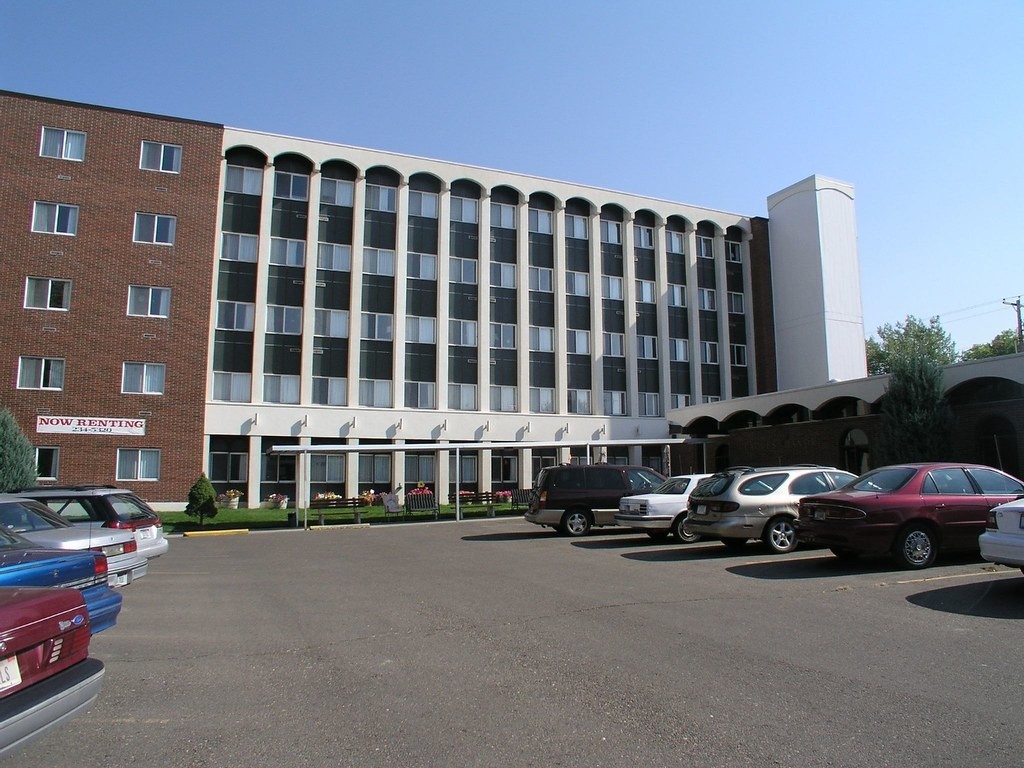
382, 494, 405, 522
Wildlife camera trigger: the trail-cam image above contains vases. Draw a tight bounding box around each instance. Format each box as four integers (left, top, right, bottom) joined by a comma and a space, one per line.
323, 501, 337, 507
368, 503, 372, 507
460, 496, 473, 506
269, 499, 288, 510
221, 497, 240, 509
499, 496, 512, 503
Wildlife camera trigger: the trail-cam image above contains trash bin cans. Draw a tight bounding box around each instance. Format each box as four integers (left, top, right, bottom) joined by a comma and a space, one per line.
288, 513, 296, 526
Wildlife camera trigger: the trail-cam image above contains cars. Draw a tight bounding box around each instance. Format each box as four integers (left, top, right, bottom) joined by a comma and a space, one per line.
0, 524, 122, 636
614, 471, 773, 545
978, 498, 1024, 574
0, 494, 147, 588
0, 586, 109, 762
793, 462, 1024, 569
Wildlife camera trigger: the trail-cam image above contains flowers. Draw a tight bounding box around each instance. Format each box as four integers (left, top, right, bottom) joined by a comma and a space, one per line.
315, 492, 342, 501
496, 491, 512, 496
218, 489, 245, 499
406, 481, 433, 496
269, 494, 290, 503
454, 490, 474, 496
359, 489, 387, 506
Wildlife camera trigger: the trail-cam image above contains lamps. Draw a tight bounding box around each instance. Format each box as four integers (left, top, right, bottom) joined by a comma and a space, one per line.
394, 418, 403, 434
439, 418, 448, 436
249, 413, 258, 431
599, 424, 606, 434
482, 420, 490, 437
347, 417, 356, 433
638, 424, 641, 435
561, 422, 570, 438
522, 422, 531, 438
299, 414, 308, 432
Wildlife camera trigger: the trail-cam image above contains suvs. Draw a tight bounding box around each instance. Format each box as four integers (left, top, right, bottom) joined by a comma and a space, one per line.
685, 464, 890, 554
524, 462, 683, 537
0, 485, 169, 560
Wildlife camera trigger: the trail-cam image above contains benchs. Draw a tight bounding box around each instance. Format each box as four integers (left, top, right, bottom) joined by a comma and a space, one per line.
512, 489, 533, 512
310, 498, 369, 526
405, 495, 441, 522
448, 491, 501, 520
